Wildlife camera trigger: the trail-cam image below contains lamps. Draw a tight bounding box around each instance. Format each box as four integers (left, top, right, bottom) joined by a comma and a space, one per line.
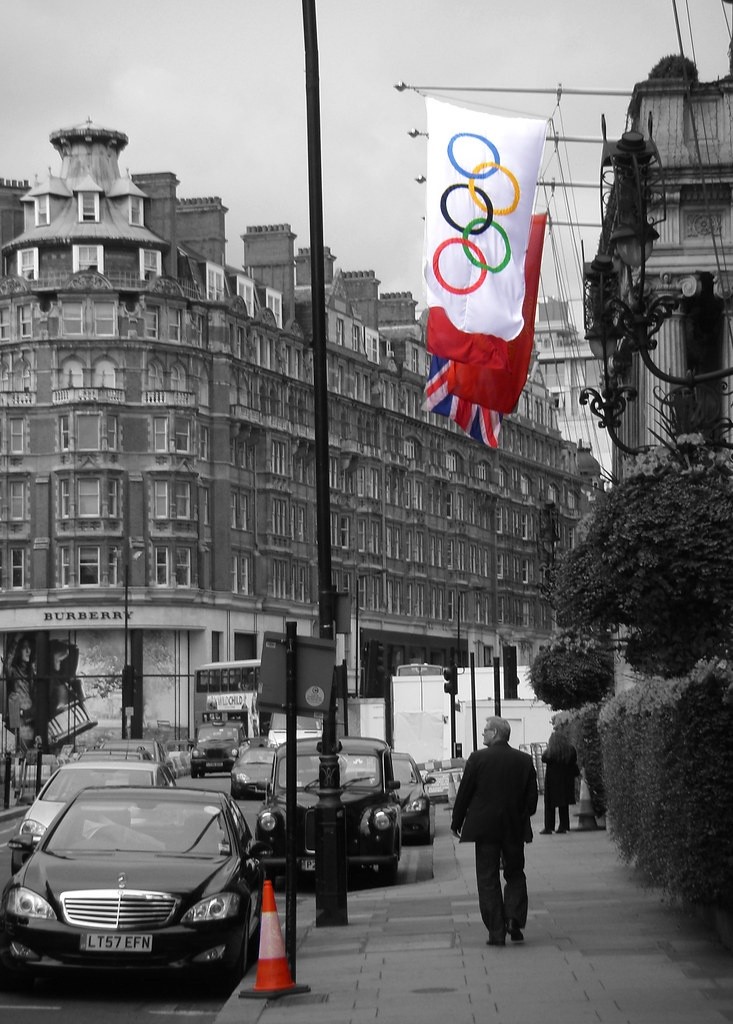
578, 111, 674, 457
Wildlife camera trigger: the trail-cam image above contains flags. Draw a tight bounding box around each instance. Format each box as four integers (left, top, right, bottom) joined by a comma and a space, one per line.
416, 98, 548, 446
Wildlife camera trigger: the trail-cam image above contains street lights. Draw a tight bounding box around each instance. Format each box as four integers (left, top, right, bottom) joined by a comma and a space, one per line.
122, 544, 144, 739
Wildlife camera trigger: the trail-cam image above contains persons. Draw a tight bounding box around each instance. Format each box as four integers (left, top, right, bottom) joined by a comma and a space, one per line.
538, 732, 584, 835
449, 716, 538, 946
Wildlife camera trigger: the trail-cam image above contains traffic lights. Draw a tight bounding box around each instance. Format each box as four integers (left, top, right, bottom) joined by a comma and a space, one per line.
364, 639, 386, 694
443, 664, 459, 695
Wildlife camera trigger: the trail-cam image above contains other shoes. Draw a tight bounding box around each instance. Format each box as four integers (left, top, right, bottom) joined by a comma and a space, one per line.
555, 828, 566, 834
507, 919, 523, 941
540, 829, 552, 834
487, 941, 505, 946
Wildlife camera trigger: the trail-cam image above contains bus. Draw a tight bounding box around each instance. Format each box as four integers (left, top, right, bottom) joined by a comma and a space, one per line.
194, 658, 272, 745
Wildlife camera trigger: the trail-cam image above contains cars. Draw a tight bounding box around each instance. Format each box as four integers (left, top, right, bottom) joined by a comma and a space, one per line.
384, 749, 437, 844
76, 744, 153, 764
230, 747, 276, 800
11, 761, 181, 875
253, 737, 403, 885
98, 739, 167, 762
427, 767, 463, 803
0, 785, 273, 991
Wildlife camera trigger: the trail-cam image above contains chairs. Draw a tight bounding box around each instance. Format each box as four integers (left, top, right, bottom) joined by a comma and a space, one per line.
63, 774, 105, 801
297, 756, 375, 786
67, 809, 220, 854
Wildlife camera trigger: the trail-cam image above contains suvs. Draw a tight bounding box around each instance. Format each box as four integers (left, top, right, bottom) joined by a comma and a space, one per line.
188, 717, 247, 777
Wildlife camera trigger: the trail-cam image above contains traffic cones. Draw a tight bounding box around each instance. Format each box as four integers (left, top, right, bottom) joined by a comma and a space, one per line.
237, 878, 312, 1000
443, 771, 457, 810
455, 774, 463, 796
572, 774, 599, 832
